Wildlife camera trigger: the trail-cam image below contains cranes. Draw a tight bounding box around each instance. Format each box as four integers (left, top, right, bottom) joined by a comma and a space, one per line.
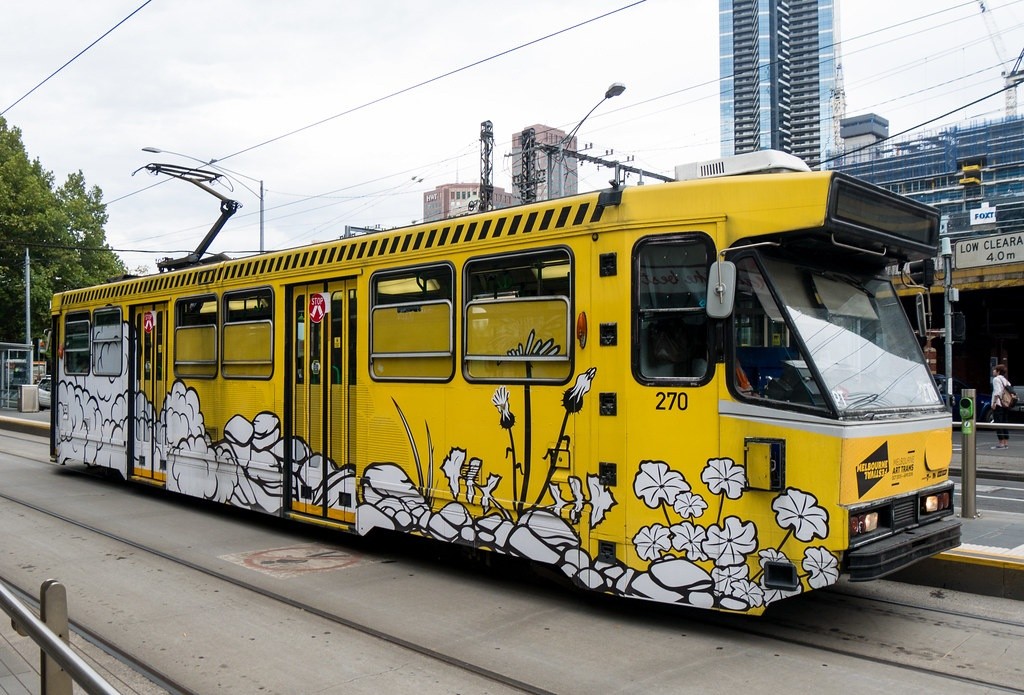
1005, 47, 1024, 119
830, 62, 849, 153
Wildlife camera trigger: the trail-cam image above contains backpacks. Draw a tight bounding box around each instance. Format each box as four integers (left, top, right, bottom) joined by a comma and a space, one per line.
997, 376, 1018, 411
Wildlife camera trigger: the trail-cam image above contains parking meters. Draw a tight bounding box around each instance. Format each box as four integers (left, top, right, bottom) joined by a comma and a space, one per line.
960, 389, 978, 520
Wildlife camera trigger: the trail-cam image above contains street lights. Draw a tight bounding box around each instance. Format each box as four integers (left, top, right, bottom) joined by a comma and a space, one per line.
558, 81, 625, 198
143, 146, 264, 254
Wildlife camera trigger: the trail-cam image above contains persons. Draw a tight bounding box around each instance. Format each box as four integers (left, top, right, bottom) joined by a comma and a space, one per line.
990, 364, 1011, 449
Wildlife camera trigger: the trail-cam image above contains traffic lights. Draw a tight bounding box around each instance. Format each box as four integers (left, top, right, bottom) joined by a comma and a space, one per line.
910, 257, 936, 288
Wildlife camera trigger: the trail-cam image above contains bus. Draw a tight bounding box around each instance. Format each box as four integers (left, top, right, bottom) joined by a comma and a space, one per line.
37, 169, 963, 617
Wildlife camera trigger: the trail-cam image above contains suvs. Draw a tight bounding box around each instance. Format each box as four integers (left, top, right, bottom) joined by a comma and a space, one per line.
38, 375, 52, 409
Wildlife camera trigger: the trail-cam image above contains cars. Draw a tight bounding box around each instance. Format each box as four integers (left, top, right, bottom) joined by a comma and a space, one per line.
931, 374, 995, 431
786, 361, 820, 395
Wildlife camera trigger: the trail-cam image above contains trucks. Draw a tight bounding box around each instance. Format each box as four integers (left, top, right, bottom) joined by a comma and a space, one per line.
30, 361, 47, 382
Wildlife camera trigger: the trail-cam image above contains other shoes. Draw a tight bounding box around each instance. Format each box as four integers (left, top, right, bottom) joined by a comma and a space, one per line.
990, 444, 1009, 449
